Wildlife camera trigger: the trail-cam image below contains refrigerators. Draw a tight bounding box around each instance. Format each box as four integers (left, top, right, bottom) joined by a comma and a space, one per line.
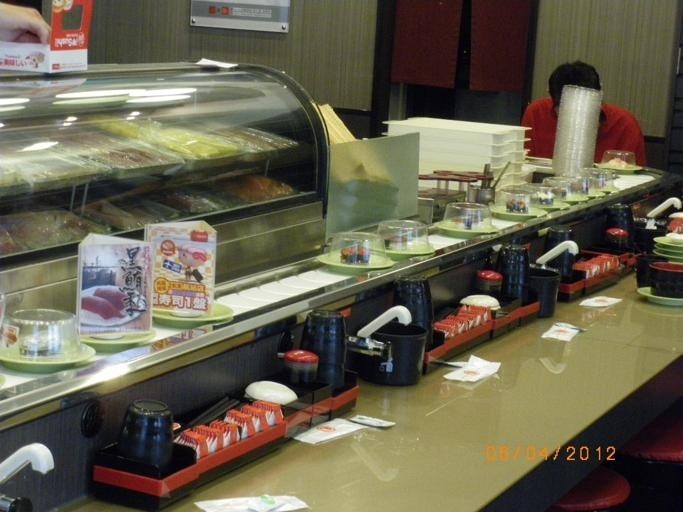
0, 59, 329, 311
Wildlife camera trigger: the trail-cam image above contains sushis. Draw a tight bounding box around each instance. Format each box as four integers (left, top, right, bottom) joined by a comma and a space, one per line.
342, 247, 370, 263
506, 183, 588, 213
461, 216, 473, 229
384, 236, 407, 250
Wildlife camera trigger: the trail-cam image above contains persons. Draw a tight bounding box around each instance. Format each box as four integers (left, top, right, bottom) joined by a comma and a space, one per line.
520, 59, 646, 167
0, 1, 53, 45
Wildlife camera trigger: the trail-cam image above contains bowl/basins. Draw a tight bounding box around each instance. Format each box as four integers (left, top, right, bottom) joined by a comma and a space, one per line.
528, 270, 560, 317
635, 223, 667, 253
354, 320, 428, 386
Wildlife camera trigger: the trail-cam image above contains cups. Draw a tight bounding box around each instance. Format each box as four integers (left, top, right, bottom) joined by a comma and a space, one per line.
301, 307, 348, 392
116, 400, 173, 466
544, 226, 576, 281
602, 204, 636, 240
392, 278, 435, 349
496, 243, 528, 305
467, 185, 495, 206
636, 255, 683, 297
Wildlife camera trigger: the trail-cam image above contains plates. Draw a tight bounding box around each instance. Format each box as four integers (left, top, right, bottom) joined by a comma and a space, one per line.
635, 287, 681, 306
434, 220, 498, 238
651, 234, 683, 262
460, 295, 501, 312
0, 303, 233, 372
243, 382, 298, 406
491, 164, 646, 237
320, 239, 437, 275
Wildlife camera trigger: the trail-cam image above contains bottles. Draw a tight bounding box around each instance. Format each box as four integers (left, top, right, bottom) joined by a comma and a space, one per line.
476, 271, 502, 296
282, 350, 318, 391
607, 228, 629, 250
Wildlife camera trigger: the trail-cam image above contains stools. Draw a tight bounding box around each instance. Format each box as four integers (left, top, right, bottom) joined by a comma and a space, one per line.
549, 466, 632, 512
620, 413, 683, 465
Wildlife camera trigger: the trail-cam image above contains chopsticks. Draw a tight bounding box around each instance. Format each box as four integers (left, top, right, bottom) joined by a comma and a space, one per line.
185, 394, 241, 432
481, 162, 509, 188
